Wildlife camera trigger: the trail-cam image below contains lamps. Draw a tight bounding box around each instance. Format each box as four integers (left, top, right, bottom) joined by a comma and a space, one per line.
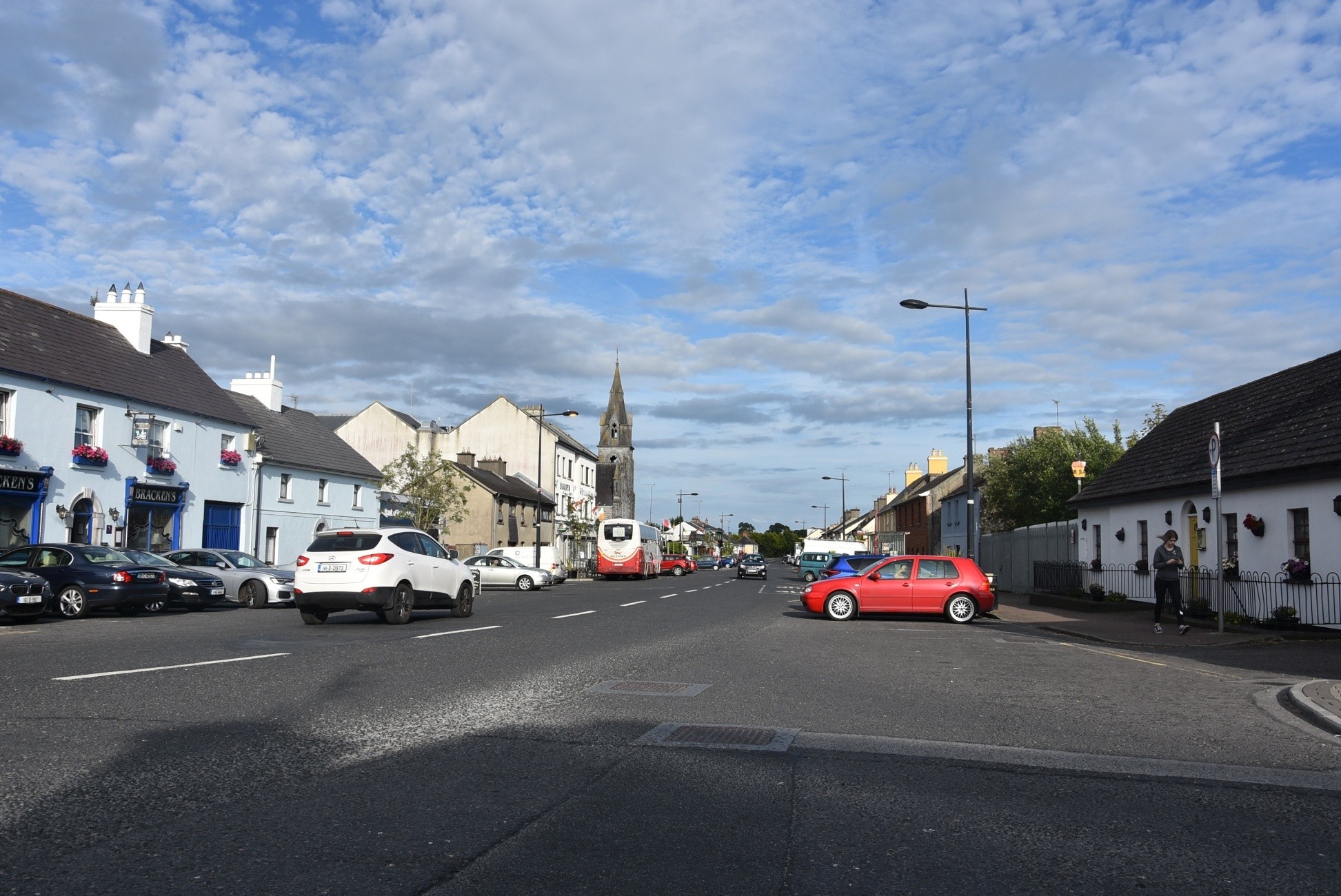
109, 507, 121, 523
1202, 506, 1211, 523
1332, 495, 1341, 516
1164, 510, 1172, 526
1081, 518, 1087, 531
55, 504, 68, 521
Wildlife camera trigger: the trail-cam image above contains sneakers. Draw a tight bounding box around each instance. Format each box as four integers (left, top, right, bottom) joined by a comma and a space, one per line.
1178, 624, 1190, 635
1153, 625, 1162, 634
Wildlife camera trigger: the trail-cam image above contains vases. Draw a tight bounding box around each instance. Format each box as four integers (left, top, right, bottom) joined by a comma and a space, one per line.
1114, 533, 1124, 541
145, 464, 174, 476
1250, 518, 1266, 538
0, 448, 20, 456
1224, 568, 1236, 579
73, 456, 107, 468
1287, 570, 1304, 580
220, 458, 238, 466
1091, 563, 1100, 568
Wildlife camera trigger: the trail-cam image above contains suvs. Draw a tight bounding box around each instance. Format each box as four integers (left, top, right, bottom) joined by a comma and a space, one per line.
799, 555, 994, 625
660, 553, 740, 577
737, 552, 767, 580
817, 554, 943, 581
293, 526, 476, 625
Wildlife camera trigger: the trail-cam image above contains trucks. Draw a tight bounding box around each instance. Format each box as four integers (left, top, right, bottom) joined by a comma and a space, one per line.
802, 539, 870, 556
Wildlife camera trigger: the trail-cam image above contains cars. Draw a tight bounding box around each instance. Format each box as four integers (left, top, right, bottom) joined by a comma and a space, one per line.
0, 542, 169, 620
781, 556, 801, 568
460, 555, 554, 591
106, 547, 226, 613
0, 567, 54, 625
269, 561, 297, 571
146, 548, 295, 610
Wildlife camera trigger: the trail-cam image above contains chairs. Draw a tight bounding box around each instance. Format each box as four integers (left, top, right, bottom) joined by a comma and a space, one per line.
217, 554, 233, 568
197, 556, 205, 566
43, 555, 57, 566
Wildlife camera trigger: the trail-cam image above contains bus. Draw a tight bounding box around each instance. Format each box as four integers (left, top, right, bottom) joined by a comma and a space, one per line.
596, 518, 664, 580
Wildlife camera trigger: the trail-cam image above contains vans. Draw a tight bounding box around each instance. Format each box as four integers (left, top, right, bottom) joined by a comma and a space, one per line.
474, 546, 565, 584
798, 552, 843, 582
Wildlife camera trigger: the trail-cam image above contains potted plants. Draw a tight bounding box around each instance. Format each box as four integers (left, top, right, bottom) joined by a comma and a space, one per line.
1135, 559, 1146, 570
1186, 596, 1212, 618
1272, 606, 1299, 626
1090, 584, 1105, 596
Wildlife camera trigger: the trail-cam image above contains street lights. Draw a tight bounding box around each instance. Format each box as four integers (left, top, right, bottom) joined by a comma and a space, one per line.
899, 288, 989, 563
676, 489, 699, 553
811, 503, 830, 539
794, 520, 806, 538
718, 511, 734, 556
822, 472, 850, 540
529, 405, 579, 568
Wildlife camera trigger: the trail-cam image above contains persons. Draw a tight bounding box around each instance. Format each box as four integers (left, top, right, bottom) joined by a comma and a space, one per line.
1153, 529, 1191, 635
491, 558, 499, 566
894, 564, 912, 578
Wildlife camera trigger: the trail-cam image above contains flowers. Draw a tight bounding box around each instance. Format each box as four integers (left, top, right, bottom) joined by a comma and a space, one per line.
1216, 513, 1309, 573
0, 435, 241, 472
1116, 530, 1123, 534
1090, 559, 1098, 565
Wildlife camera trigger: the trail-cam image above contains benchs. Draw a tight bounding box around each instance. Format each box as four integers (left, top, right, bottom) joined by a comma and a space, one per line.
84, 553, 119, 563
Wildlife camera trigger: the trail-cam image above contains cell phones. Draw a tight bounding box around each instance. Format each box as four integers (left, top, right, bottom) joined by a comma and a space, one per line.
1174, 557, 1179, 560
